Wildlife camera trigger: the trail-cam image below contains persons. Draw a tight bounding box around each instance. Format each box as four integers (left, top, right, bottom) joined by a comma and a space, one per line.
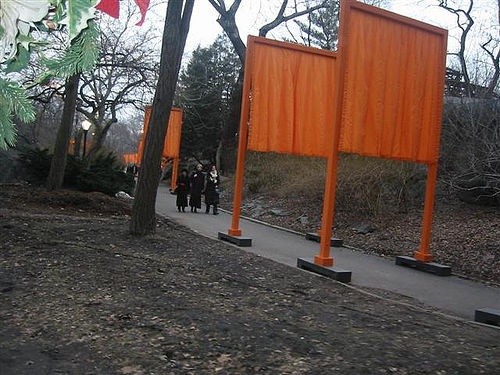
204, 164, 220, 215
126, 164, 138, 176
189, 163, 205, 213
176, 169, 190, 212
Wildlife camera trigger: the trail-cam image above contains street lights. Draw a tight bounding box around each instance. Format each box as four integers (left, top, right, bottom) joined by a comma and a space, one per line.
81, 119, 93, 157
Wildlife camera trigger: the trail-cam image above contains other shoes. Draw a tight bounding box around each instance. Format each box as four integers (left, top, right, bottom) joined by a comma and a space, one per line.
205, 210, 209, 213
213, 212, 219, 214
191, 209, 197, 213
178, 209, 186, 212
